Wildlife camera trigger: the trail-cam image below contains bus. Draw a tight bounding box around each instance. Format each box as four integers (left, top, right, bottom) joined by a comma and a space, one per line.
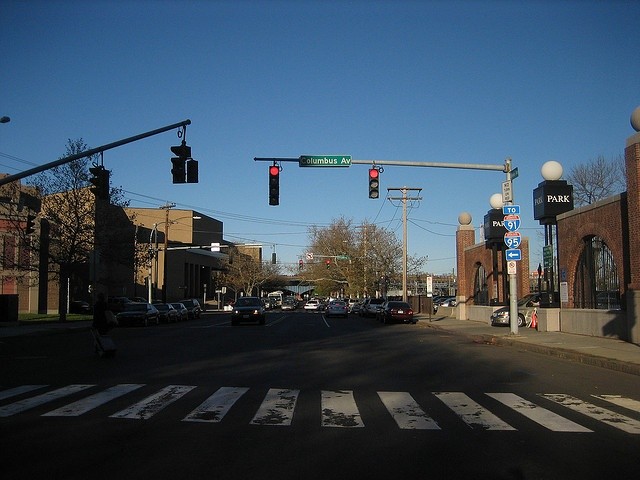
268, 291, 284, 306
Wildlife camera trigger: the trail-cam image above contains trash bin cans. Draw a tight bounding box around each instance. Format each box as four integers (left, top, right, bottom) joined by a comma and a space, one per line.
0, 294, 19, 322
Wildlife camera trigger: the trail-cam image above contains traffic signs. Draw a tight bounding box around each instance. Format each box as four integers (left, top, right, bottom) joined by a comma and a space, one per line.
504, 232, 522, 249
509, 167, 518, 180
501, 179, 512, 202
543, 245, 553, 268
504, 215, 521, 231
306, 252, 313, 260
337, 254, 351, 260
299, 154, 352, 168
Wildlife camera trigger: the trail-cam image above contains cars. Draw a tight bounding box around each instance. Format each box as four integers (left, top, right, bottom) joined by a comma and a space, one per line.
376, 301, 387, 320
154, 304, 177, 322
432, 295, 455, 313
348, 297, 381, 319
304, 298, 325, 311
281, 296, 300, 310
378, 301, 413, 324
325, 301, 347, 318
116, 303, 161, 326
261, 298, 276, 310
171, 303, 188, 321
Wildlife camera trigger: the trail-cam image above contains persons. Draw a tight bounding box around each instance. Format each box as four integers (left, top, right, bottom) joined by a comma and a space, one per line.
92, 293, 110, 330
530, 296, 541, 307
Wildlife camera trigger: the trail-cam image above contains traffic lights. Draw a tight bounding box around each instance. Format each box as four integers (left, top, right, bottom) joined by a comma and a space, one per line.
299, 260, 303, 271
268, 166, 280, 206
326, 260, 330, 270
369, 168, 380, 199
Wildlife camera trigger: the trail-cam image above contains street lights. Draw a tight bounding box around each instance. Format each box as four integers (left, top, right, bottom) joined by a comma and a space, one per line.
147, 220, 178, 304
167, 216, 202, 228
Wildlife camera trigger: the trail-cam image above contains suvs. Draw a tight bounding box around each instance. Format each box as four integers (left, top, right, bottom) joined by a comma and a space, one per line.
231, 296, 267, 325
180, 298, 202, 320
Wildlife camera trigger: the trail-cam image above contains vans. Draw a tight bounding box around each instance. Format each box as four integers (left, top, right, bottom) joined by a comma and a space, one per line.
491, 292, 559, 327
